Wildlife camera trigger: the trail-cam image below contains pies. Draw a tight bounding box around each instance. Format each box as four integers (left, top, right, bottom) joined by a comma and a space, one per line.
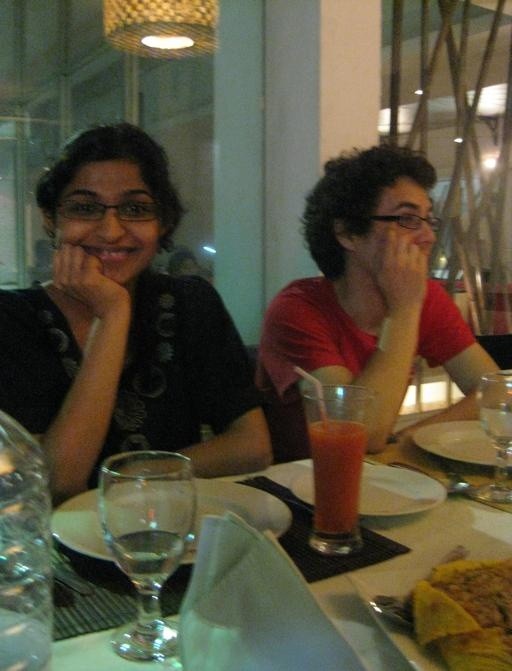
411, 559, 511, 670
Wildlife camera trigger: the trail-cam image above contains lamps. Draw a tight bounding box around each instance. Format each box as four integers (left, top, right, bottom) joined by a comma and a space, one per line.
103, 0, 217, 58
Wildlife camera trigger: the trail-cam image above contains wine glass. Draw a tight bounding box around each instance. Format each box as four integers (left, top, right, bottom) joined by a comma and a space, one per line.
294, 379, 374, 559
96, 445, 198, 662
464, 370, 511, 502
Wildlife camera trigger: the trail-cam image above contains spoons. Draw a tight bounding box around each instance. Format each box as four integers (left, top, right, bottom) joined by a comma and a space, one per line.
357, 544, 471, 627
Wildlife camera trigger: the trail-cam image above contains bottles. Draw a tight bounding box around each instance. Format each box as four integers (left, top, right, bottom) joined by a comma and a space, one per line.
1, 408, 54, 671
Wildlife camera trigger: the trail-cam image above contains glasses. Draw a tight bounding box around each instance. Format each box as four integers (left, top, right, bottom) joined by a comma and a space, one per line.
55, 200, 157, 222
350, 213, 443, 232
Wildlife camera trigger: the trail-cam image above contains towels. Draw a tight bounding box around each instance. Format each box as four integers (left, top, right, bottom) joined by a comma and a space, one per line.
179, 507, 367, 671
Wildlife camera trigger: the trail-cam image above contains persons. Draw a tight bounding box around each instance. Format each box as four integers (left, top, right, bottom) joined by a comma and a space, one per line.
0, 121, 276, 512
256, 142, 510, 466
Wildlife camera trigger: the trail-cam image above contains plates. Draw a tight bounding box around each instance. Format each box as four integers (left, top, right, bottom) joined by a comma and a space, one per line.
412, 417, 498, 467
344, 566, 435, 669
51, 471, 293, 564
284, 463, 446, 521
